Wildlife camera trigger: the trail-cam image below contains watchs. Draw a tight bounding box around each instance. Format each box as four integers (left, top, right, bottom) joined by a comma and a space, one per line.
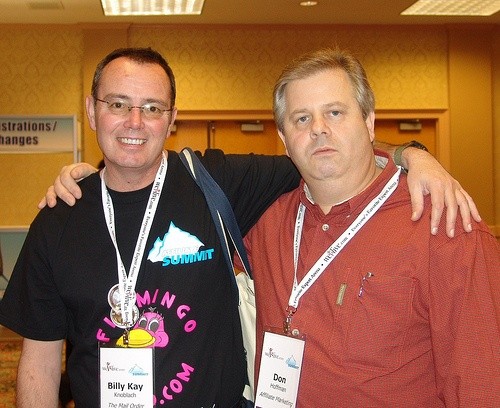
393, 140, 429, 175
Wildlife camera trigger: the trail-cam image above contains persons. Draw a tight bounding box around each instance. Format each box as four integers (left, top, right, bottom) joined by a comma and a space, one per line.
0, 45, 484, 408
39, 51, 500, 408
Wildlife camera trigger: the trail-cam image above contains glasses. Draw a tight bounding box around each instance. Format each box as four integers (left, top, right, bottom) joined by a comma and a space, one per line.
94, 96, 174, 122
